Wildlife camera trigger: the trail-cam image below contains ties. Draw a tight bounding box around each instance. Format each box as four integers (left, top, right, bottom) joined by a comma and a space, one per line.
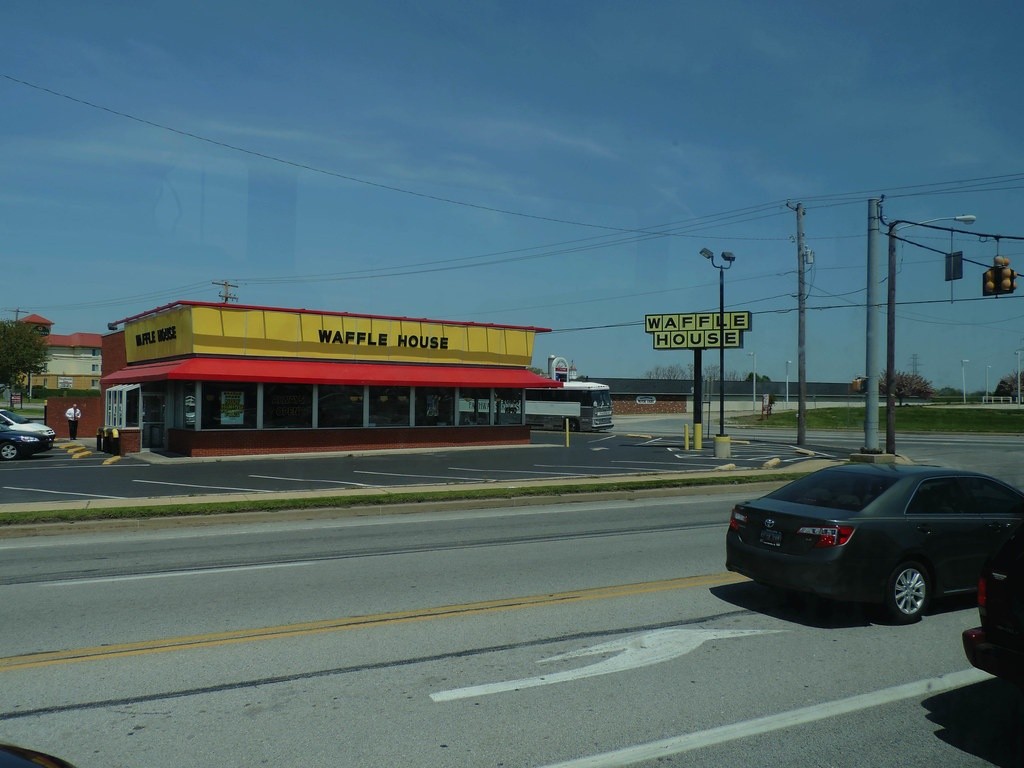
74, 411, 75, 420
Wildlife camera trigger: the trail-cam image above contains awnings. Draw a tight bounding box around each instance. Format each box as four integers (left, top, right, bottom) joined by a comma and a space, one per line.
100, 358, 564, 388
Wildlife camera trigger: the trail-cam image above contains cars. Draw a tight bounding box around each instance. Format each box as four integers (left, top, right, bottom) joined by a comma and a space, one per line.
960, 526, 1024, 688
723, 460, 1024, 625
0, 423, 52, 460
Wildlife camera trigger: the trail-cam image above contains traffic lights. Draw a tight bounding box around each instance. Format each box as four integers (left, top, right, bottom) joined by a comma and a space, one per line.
982, 255, 1018, 297
850, 376, 864, 394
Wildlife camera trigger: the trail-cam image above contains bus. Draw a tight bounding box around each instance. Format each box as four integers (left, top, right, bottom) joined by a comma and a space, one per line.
459, 380, 617, 434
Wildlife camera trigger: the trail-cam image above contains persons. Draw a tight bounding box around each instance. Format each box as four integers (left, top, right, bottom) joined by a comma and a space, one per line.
65, 404, 81, 440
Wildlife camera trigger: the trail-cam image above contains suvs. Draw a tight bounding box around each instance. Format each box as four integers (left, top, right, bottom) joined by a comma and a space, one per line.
0, 408, 57, 442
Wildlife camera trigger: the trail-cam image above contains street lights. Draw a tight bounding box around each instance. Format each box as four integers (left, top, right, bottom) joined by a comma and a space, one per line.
986, 364, 993, 404
1014, 351, 1021, 405
746, 351, 757, 417
699, 247, 737, 438
960, 359, 969, 404
883, 213, 978, 454
784, 359, 793, 411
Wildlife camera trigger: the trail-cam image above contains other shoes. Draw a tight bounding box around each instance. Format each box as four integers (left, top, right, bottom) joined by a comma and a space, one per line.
70, 438, 78, 440
